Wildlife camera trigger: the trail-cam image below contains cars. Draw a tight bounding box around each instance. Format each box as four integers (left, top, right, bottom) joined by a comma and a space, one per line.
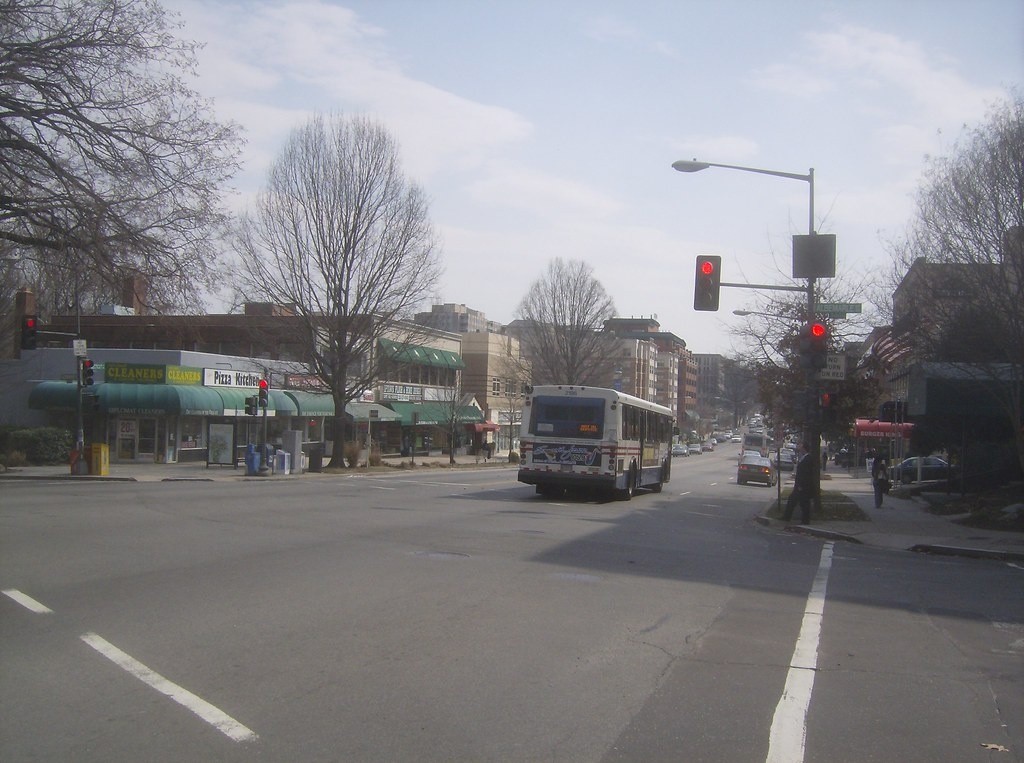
705, 413, 799, 464
671, 444, 690, 457
689, 444, 702, 455
737, 451, 761, 465
738, 457, 777, 487
774, 453, 794, 470
887, 456, 955, 484
701, 442, 714, 452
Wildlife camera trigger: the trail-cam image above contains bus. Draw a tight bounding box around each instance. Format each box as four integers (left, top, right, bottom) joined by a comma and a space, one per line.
741, 432, 771, 459
517, 383, 679, 501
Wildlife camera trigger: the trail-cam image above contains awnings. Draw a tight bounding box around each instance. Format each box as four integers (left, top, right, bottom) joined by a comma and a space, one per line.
28, 381, 336, 424
345, 402, 500, 432
379, 338, 466, 369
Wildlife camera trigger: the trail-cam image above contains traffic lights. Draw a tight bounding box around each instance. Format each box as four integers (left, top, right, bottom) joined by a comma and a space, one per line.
259, 379, 268, 407
245, 396, 255, 415
21, 315, 38, 350
694, 254, 722, 311
810, 321, 827, 368
83, 359, 94, 386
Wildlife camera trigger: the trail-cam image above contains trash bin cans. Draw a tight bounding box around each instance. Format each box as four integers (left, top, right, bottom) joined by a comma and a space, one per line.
245, 443, 274, 476
301, 450, 305, 474
276, 449, 290, 475
92, 443, 109, 476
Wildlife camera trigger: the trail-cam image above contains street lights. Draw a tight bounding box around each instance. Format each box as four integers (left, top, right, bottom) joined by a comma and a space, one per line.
671, 159, 821, 507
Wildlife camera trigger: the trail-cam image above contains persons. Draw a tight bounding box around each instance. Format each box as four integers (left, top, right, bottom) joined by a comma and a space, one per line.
777, 441, 813, 525
872, 455, 889, 508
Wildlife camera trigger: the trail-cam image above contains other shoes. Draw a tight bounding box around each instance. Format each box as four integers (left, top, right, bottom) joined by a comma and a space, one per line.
776, 516, 791, 521
798, 520, 809, 525
